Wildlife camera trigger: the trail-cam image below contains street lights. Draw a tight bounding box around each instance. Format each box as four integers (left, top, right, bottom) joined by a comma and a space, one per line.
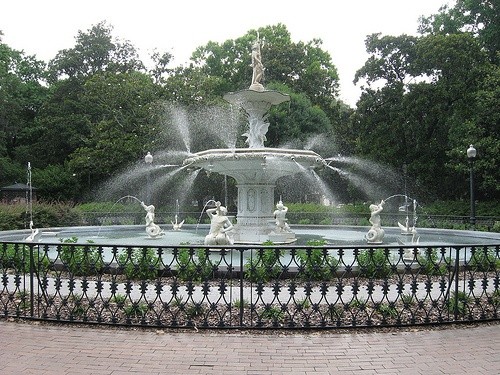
143, 151, 152, 205
348, 172, 355, 206
466, 144, 478, 226
400, 160, 408, 197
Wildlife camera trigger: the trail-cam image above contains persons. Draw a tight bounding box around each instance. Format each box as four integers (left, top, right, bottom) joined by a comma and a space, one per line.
367, 200, 386, 243
140, 201, 162, 236
205, 199, 234, 245
274, 201, 288, 232
248, 38, 265, 85
